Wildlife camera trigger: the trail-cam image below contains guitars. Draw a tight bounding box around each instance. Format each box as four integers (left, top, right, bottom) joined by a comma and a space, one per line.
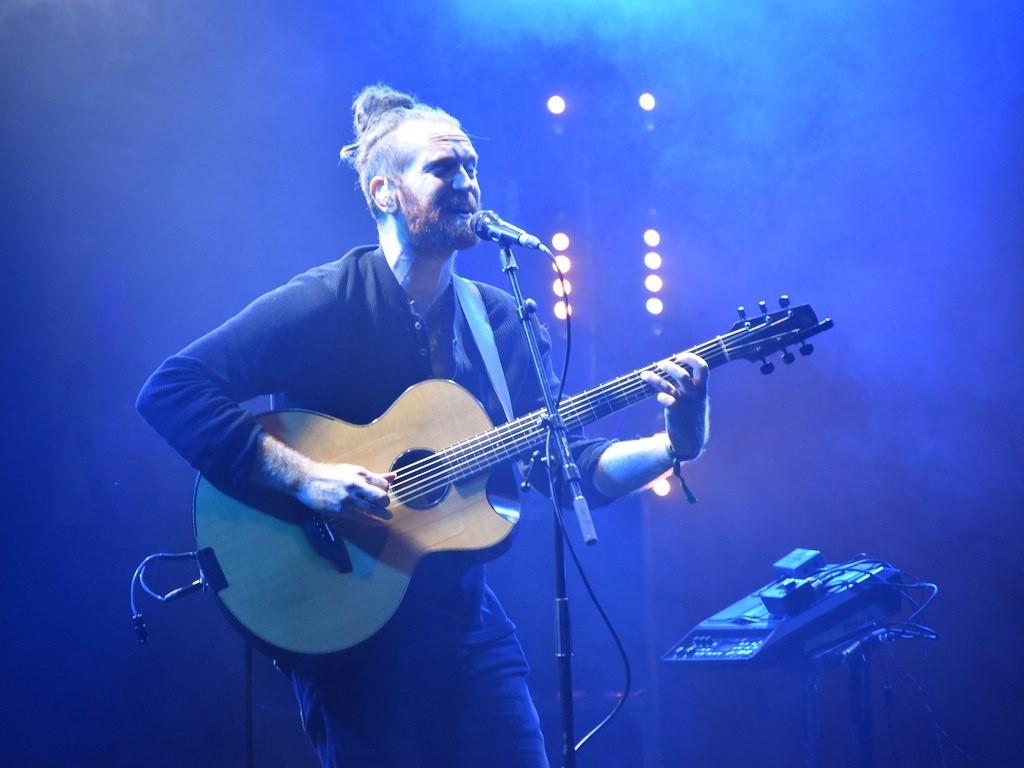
185, 294, 839, 680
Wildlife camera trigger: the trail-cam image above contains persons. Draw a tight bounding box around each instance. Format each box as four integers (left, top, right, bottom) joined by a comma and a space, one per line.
132, 85, 715, 768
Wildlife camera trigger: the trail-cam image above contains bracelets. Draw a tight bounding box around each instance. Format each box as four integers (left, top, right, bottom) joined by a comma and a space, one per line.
662, 422, 705, 505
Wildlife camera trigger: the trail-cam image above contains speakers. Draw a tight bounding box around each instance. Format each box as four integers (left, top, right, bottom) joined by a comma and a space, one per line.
653, 561, 918, 768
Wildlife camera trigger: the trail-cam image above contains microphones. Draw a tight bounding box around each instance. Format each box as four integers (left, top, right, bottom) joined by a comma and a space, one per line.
839, 629, 899, 668
469, 209, 542, 249
